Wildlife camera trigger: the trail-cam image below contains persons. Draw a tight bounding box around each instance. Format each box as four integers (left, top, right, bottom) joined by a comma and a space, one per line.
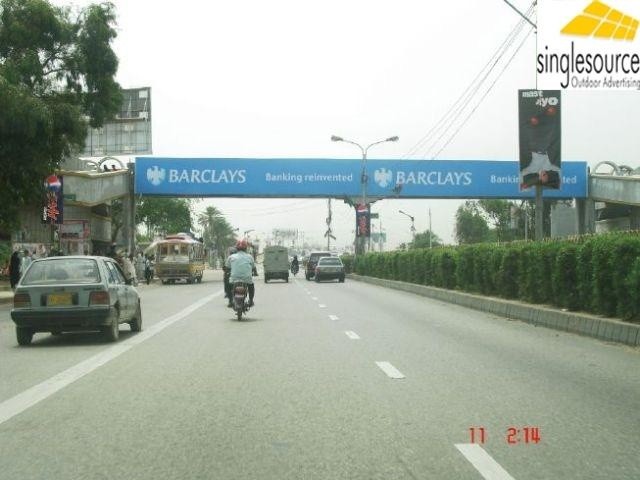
521, 106, 561, 190
9, 244, 156, 291
291, 255, 299, 273
223, 240, 258, 308
222, 247, 237, 298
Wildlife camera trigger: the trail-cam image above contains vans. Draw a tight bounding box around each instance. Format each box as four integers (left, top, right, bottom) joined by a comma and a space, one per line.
305, 252, 331, 281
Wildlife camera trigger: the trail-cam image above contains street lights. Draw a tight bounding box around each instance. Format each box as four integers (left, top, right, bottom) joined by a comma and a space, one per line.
330, 134, 400, 205
232, 228, 255, 237
399, 210, 414, 248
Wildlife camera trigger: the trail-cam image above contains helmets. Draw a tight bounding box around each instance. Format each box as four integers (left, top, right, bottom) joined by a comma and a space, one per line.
235, 239, 248, 250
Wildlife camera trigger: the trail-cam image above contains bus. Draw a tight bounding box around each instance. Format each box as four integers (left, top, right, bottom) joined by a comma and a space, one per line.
156, 235, 205, 284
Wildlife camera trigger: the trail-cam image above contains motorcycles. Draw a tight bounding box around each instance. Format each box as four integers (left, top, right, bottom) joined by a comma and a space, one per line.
291, 265, 299, 276
231, 279, 250, 321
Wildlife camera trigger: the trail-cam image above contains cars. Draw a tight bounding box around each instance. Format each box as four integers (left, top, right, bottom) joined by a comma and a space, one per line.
10, 255, 142, 346
314, 256, 346, 282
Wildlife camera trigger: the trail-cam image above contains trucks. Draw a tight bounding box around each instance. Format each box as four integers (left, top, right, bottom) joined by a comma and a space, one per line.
264, 245, 289, 283
